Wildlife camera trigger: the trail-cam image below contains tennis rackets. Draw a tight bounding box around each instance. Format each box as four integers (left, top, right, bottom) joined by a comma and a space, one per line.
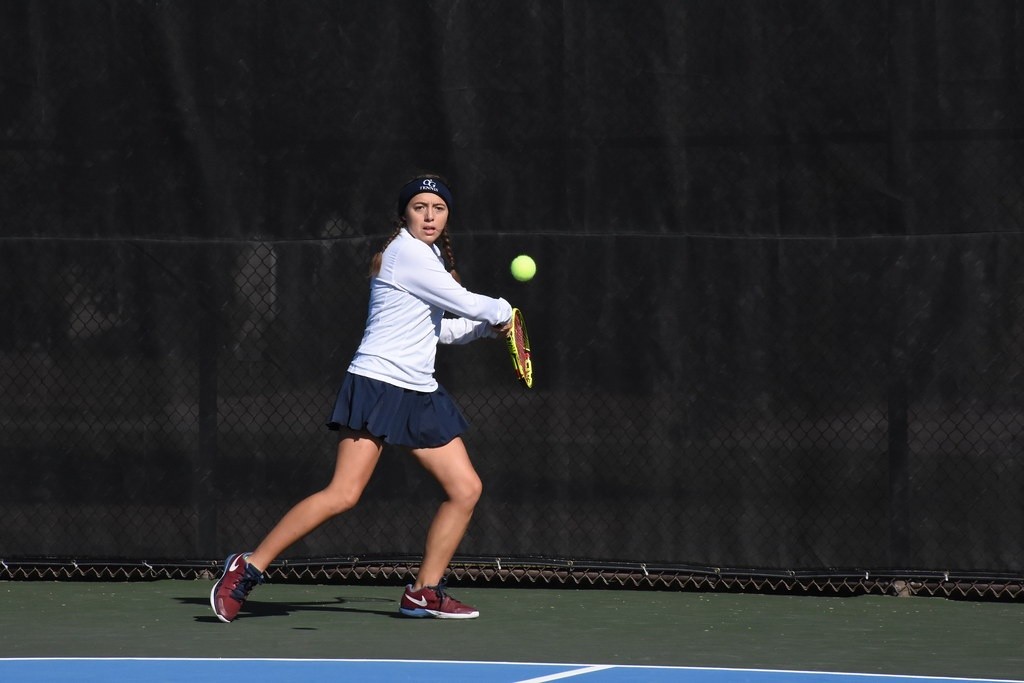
495, 308, 533, 392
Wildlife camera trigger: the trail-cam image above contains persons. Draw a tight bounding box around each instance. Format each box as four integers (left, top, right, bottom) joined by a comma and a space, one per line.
210, 174, 513, 624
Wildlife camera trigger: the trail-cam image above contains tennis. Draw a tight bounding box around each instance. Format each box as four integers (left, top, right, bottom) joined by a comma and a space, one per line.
511, 254, 536, 282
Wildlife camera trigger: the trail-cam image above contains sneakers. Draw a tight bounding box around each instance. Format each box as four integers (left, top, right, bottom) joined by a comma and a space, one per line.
209, 551, 272, 623
400, 577, 479, 619
